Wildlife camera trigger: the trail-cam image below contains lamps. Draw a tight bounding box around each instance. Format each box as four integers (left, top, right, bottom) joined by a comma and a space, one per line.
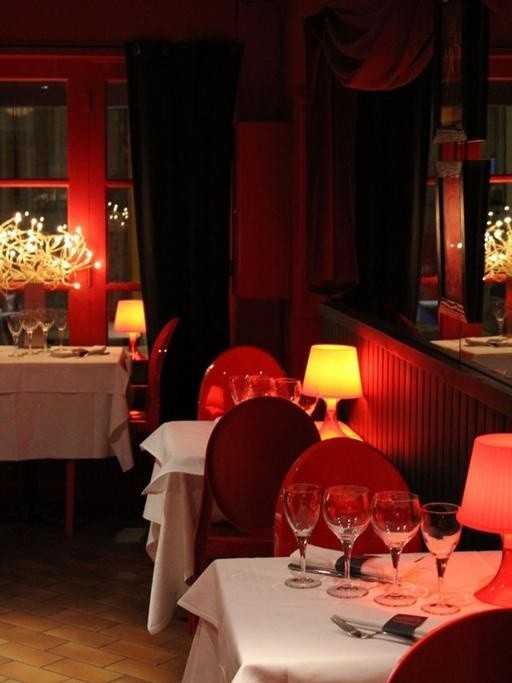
301, 344, 362, 441
0, 211, 100, 292
113, 298, 148, 361
455, 433, 512, 607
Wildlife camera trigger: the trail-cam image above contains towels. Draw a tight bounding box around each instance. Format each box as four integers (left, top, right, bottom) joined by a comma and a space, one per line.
290, 544, 417, 583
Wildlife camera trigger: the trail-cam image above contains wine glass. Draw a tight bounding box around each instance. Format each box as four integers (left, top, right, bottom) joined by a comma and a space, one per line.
280, 483, 326, 589
7, 307, 68, 357
321, 485, 370, 601
489, 300, 508, 342
418, 500, 464, 617
369, 489, 424, 608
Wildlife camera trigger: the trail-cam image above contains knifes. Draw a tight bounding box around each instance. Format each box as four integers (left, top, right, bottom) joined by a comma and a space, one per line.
287, 559, 401, 591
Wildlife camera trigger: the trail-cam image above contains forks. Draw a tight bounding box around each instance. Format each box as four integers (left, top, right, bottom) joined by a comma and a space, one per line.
327, 613, 420, 648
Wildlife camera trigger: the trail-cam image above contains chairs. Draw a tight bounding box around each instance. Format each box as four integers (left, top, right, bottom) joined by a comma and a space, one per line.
385, 606, 512, 683
273, 436, 423, 556
65, 319, 178, 537
204, 396, 321, 548
197, 344, 291, 422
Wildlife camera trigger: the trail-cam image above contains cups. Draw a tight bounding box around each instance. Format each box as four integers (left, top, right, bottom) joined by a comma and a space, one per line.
225, 373, 301, 407
